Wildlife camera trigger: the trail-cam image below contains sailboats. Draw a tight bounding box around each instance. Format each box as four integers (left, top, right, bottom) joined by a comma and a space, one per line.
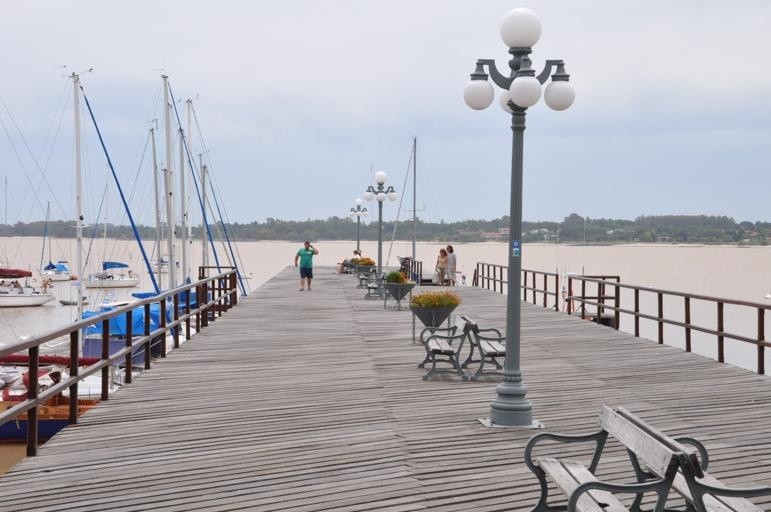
39, 200, 72, 281
73, 73, 252, 368
0, 176, 33, 279
0, 67, 176, 439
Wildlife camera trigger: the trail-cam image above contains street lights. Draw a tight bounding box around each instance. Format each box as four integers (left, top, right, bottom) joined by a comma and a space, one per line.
350, 171, 398, 277
462, 7, 575, 429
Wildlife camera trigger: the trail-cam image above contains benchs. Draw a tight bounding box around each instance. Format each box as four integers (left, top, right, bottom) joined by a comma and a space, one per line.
356, 268, 388, 297
526, 404, 704, 512
462, 315, 507, 381
613, 406, 771, 512
420, 315, 470, 382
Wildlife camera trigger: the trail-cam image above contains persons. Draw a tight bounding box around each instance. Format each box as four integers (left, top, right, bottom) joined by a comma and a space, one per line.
295, 240, 319, 291
335, 249, 362, 275
446, 245, 456, 286
435, 248, 449, 286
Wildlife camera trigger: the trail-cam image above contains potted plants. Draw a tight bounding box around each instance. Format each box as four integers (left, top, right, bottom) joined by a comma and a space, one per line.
350, 257, 376, 283
410, 291, 461, 345
382, 270, 417, 311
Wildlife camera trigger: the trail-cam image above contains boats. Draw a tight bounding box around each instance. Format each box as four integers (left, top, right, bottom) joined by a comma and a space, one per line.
59, 298, 90, 307
0, 264, 56, 307
